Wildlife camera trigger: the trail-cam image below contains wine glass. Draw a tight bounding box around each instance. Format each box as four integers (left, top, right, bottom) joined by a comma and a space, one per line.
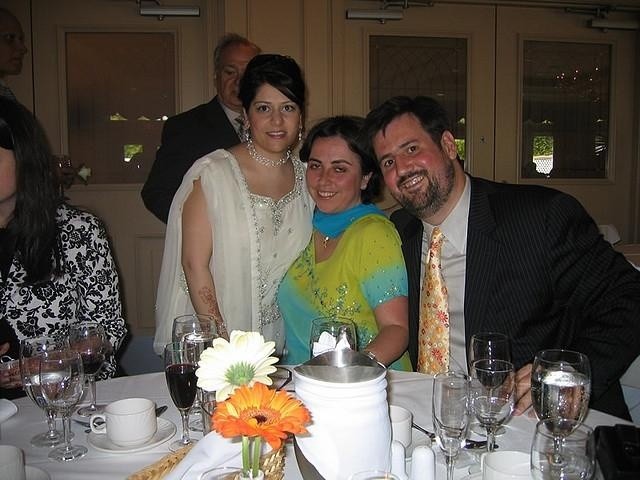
173, 314, 219, 431
21, 334, 75, 448
432, 332, 597, 480
70, 322, 111, 416
41, 343, 85, 459
163, 341, 202, 454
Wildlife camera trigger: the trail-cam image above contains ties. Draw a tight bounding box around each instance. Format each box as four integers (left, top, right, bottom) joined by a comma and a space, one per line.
235, 115, 250, 143
417, 227, 450, 377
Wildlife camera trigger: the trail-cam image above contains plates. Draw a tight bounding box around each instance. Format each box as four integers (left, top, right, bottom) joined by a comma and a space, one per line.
404, 427, 431, 461
23, 464, 49, 480
86, 416, 177, 454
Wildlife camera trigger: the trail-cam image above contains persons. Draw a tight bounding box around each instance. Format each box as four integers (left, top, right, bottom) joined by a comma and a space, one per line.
277, 114, 414, 372
0, 96, 130, 400
359, 95, 640, 423
140, 32, 261, 225
154, 55, 317, 358
0, 7, 26, 101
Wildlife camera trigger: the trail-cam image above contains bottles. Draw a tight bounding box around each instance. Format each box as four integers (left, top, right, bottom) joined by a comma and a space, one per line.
411, 446, 436, 480
389, 440, 408, 480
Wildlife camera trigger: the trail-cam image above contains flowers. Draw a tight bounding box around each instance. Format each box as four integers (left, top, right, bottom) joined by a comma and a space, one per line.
196, 327, 313, 476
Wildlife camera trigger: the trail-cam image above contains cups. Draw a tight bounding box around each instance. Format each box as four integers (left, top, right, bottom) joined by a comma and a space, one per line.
308, 316, 359, 359
88, 397, 158, 447
389, 405, 413, 448
0, 444, 27, 480
200, 467, 245, 480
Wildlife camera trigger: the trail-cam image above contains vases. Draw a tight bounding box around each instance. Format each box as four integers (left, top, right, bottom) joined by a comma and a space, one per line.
237, 469, 266, 479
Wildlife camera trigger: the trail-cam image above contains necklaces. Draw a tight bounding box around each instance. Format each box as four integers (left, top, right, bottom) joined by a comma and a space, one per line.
246, 139, 291, 167
319, 232, 329, 248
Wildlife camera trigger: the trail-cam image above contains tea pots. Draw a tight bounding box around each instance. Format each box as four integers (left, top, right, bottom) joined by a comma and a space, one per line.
291, 327, 392, 480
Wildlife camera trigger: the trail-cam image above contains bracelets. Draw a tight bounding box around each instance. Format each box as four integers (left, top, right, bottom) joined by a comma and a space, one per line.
218, 324, 226, 335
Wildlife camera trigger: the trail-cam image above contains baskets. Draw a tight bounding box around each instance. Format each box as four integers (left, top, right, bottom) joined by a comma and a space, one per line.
128, 437, 286, 480
